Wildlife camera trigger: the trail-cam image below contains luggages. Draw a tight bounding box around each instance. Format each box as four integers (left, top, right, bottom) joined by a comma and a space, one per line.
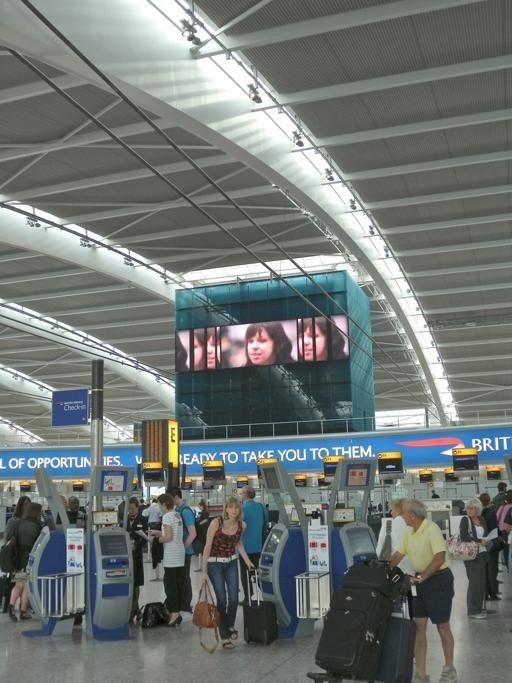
243, 567, 277, 646
314, 559, 415, 682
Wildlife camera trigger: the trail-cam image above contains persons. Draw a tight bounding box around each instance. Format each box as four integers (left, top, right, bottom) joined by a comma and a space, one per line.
368, 500, 388, 515
193, 325, 232, 371
298, 315, 345, 362
376, 498, 416, 576
388, 500, 457, 683
244, 321, 296, 367
432, 489, 440, 498
0, 486, 269, 649
459, 482, 512, 619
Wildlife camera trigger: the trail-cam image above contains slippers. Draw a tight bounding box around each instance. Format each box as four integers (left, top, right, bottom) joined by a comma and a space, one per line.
223, 640, 234, 649
229, 627, 238, 640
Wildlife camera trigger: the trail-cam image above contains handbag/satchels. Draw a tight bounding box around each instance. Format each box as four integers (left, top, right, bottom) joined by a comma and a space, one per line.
379, 535, 392, 561
142, 602, 170, 627
446, 535, 478, 561
193, 601, 222, 628
1, 527, 19, 573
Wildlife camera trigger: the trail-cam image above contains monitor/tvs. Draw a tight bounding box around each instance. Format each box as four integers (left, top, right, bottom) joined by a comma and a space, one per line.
379, 455, 504, 482
237, 481, 248, 488
345, 529, 376, 553
318, 478, 332, 487
72, 484, 84, 493
144, 469, 162, 480
325, 463, 338, 476
181, 482, 192, 490
20, 485, 32, 492
347, 466, 368, 486
100, 534, 129, 556
203, 467, 225, 480
263, 529, 284, 552
295, 479, 307, 487
264, 468, 281, 489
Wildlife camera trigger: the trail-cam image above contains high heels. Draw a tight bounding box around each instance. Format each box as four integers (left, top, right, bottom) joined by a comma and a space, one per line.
166, 615, 182, 627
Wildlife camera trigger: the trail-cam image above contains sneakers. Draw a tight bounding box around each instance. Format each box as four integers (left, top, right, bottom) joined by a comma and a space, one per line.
438, 666, 456, 683
410, 676, 430, 683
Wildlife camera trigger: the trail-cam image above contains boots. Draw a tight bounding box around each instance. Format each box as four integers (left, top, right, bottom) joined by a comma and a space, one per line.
9, 604, 32, 621
